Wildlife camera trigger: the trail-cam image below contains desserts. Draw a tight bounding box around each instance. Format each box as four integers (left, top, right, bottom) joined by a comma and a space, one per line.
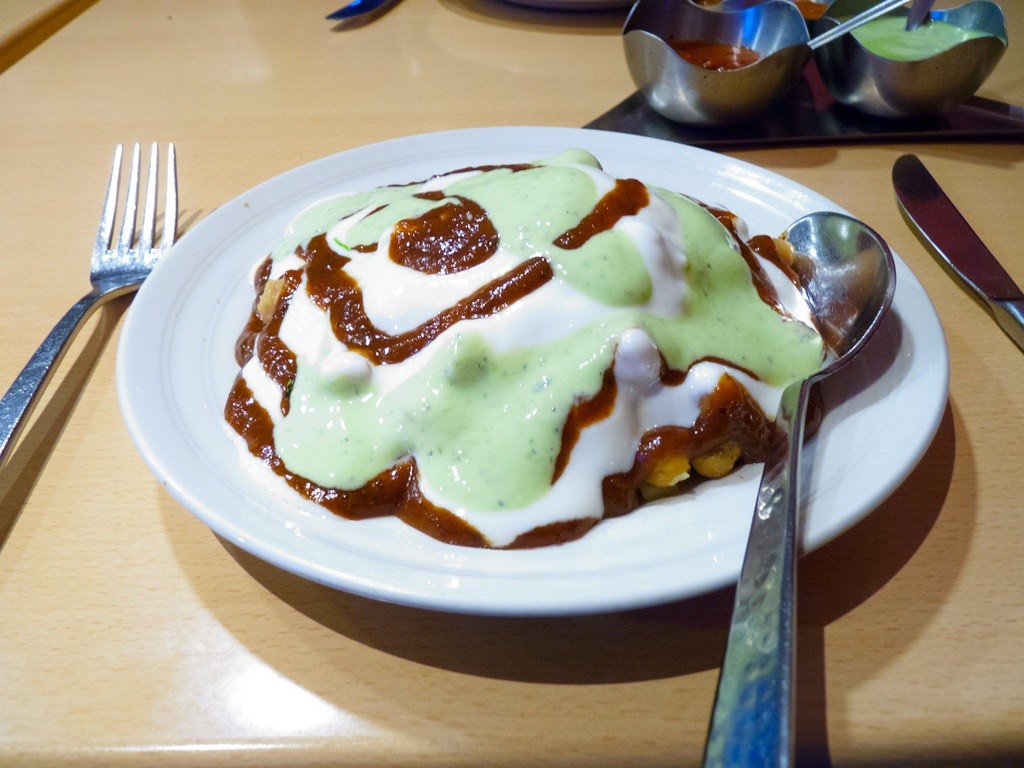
220, 145, 833, 551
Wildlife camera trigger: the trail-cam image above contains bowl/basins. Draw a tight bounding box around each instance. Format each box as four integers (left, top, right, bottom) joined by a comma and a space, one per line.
816, 1, 1008, 120
621, 0, 812, 128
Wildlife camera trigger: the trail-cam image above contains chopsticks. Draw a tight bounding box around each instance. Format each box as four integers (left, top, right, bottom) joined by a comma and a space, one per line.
806, 0, 911, 50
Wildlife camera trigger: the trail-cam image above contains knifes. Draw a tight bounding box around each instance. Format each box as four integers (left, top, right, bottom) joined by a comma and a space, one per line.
323, 0, 392, 20
892, 153, 1024, 352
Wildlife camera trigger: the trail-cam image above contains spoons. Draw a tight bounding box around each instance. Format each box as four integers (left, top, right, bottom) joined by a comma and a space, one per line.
699, 211, 897, 768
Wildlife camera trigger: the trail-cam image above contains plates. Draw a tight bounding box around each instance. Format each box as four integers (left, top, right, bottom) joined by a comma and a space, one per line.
113, 126, 948, 619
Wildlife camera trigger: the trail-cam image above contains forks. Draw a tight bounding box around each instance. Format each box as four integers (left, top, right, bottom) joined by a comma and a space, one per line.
0, 142, 178, 467
